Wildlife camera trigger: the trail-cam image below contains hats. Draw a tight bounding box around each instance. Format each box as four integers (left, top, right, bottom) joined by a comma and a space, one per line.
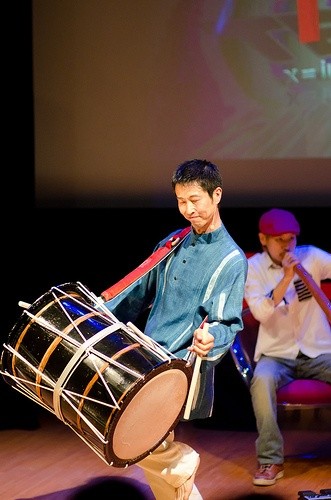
258, 209, 298, 237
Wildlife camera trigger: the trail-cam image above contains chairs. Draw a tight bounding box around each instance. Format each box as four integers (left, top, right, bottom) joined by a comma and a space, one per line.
230, 252, 331, 410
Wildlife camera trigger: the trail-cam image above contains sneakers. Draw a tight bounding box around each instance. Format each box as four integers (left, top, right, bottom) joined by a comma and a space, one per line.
251, 461, 284, 486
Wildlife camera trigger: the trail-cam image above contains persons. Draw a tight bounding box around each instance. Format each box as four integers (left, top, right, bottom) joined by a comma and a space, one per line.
89, 158, 249, 500
243, 209, 331, 487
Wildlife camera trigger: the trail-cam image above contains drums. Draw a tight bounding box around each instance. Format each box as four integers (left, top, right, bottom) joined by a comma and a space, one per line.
1, 281, 192, 467
